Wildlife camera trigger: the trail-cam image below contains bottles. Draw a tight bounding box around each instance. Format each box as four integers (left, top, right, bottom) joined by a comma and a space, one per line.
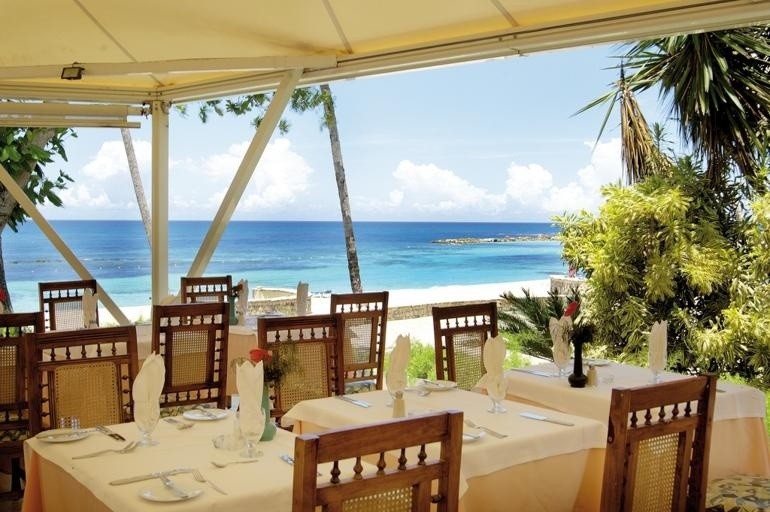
586, 364, 598, 388
238, 311, 245, 326
392, 391, 408, 420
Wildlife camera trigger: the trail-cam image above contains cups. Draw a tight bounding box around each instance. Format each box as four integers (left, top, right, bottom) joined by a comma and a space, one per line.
385, 371, 408, 401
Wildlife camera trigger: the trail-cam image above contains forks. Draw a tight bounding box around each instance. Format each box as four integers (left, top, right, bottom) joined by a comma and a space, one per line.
211, 459, 258, 468
72, 441, 139, 460
194, 469, 229, 496
465, 419, 508, 439
164, 417, 196, 430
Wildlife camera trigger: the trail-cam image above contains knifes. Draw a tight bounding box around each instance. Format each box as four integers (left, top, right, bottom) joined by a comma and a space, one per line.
512, 368, 550, 377
110, 468, 194, 485
520, 411, 575, 426
159, 474, 188, 498
95, 424, 126, 441
337, 396, 369, 408
279, 454, 294, 464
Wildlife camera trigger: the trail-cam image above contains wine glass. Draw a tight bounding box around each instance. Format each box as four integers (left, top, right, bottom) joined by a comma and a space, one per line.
240, 405, 267, 460
647, 352, 667, 384
552, 348, 570, 377
133, 402, 161, 446
484, 374, 507, 414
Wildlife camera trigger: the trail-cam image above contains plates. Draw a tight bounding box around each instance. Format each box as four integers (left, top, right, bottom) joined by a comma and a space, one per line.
425, 379, 458, 392
182, 408, 227, 421
587, 360, 610, 366
140, 482, 203, 502
36, 428, 88, 443
